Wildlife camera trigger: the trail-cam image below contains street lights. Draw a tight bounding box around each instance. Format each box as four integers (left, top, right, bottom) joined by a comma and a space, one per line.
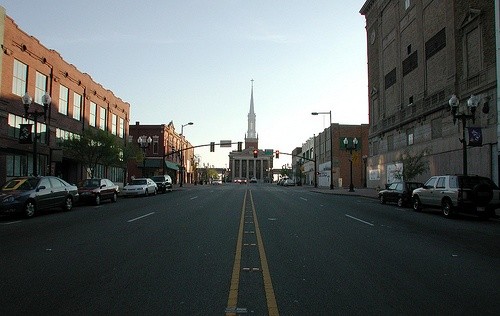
179, 122, 194, 187
343, 137, 358, 192
138, 136, 152, 178
311, 110, 335, 189
449, 93, 479, 176
22, 92, 52, 177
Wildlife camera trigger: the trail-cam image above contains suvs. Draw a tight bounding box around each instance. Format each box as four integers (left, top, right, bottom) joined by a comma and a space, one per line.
152, 175, 173, 193
410, 174, 499, 218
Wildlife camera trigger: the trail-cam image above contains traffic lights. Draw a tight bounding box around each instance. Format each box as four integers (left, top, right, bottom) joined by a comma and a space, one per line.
253, 149, 258, 158
276, 150, 279, 158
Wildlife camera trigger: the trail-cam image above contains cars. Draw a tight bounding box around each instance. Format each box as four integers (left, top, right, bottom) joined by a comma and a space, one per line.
0, 177, 80, 220
234, 177, 247, 184
122, 178, 158, 196
283, 179, 295, 186
377, 181, 424, 208
0, 177, 27, 194
75, 178, 120, 206
277, 177, 290, 186
250, 178, 257, 183
